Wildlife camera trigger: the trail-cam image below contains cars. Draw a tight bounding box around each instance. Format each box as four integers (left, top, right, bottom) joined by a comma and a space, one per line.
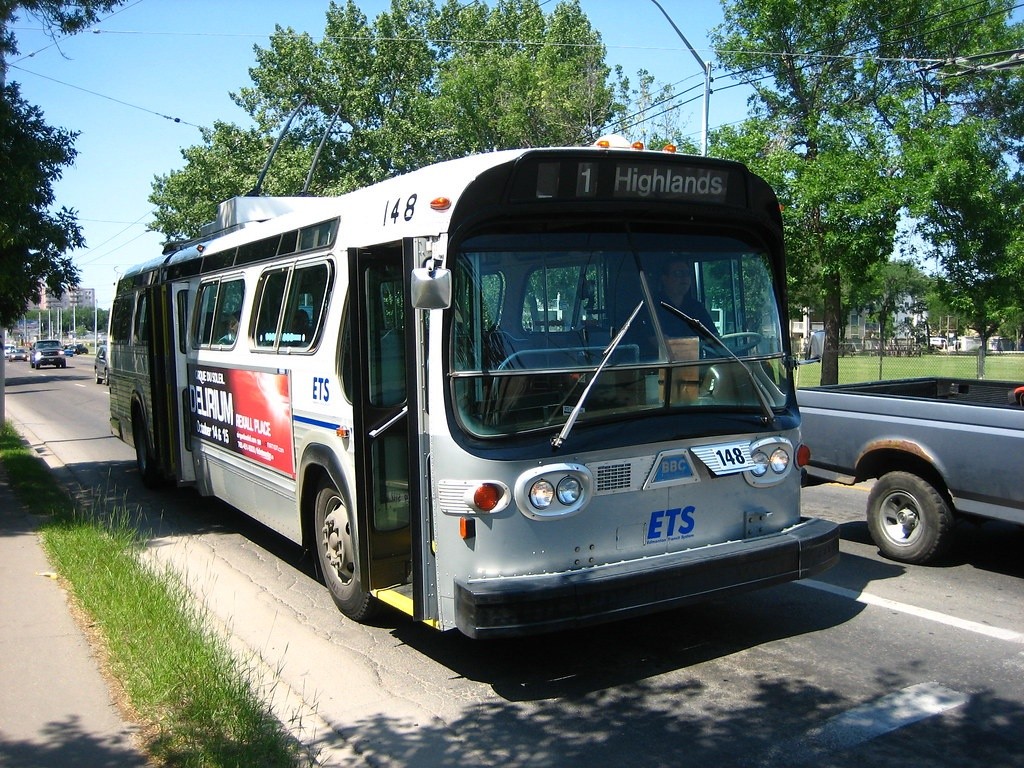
9, 348, 28, 362
64, 344, 89, 357
94, 346, 111, 386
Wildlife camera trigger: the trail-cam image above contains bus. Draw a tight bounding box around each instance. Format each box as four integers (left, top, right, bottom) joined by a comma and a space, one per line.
98, 96, 843, 645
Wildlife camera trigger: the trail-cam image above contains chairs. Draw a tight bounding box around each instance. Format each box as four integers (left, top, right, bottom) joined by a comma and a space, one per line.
376, 329, 535, 375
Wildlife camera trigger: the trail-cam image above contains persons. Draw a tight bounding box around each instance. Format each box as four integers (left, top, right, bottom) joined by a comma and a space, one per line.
292, 309, 312, 340
216, 311, 241, 344
636, 255, 725, 371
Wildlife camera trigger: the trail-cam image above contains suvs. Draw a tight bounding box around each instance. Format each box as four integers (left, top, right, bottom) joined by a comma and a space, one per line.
29, 340, 67, 370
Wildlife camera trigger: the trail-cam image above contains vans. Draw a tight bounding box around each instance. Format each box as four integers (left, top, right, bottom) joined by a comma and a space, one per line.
4, 344, 16, 357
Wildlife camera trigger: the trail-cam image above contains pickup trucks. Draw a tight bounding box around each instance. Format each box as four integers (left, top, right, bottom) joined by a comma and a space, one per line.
790, 376, 1024, 563
